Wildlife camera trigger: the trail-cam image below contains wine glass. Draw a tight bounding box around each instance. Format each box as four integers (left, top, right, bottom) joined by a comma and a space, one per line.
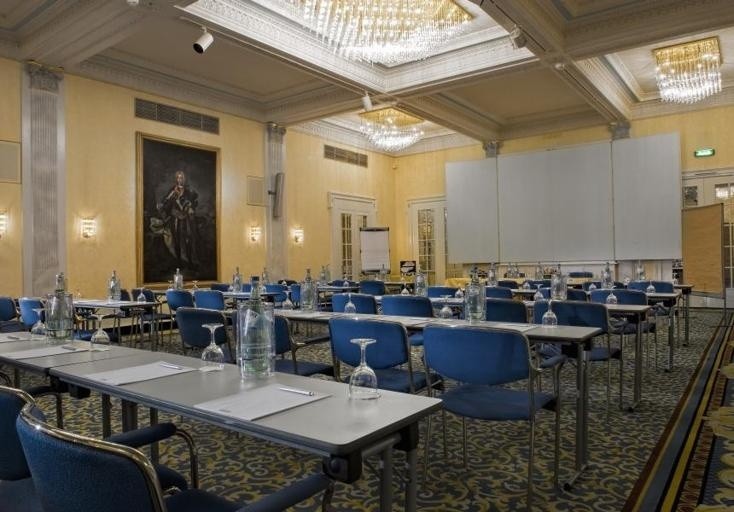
29, 306, 49, 343
88, 312, 113, 352
347, 336, 380, 402
670, 272, 679, 286
538, 277, 656, 329
137, 287, 149, 303
190, 280, 200, 295
276, 272, 411, 312
200, 323, 226, 367
431, 279, 544, 322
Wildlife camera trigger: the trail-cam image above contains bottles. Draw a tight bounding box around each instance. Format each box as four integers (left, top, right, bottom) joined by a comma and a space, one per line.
466, 266, 485, 318
109, 271, 119, 301
242, 275, 271, 377
50, 274, 68, 338
487, 260, 544, 286
303, 265, 387, 279
601, 262, 614, 289
552, 261, 567, 300
175, 266, 269, 290
635, 258, 645, 278
417, 268, 425, 294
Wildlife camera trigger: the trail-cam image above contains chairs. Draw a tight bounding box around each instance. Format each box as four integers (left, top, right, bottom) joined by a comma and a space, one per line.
0, 270, 694, 511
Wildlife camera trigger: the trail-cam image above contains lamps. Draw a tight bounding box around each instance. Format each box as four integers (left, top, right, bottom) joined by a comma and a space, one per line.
284, 0, 472, 66
652, 36, 723, 103
192, 26, 213, 54
361, 92, 372, 112
291, 224, 306, 245
79, 214, 99, 239
359, 108, 425, 152
246, 221, 263, 244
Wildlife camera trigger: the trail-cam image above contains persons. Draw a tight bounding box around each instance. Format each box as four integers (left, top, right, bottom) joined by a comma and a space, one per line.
161, 172, 201, 273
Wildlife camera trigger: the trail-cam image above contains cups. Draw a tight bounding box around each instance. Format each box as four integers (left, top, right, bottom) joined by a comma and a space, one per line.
237, 303, 280, 381
42, 292, 74, 346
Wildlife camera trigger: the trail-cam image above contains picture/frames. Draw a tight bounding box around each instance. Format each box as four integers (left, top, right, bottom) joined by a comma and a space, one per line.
135, 130, 222, 290
